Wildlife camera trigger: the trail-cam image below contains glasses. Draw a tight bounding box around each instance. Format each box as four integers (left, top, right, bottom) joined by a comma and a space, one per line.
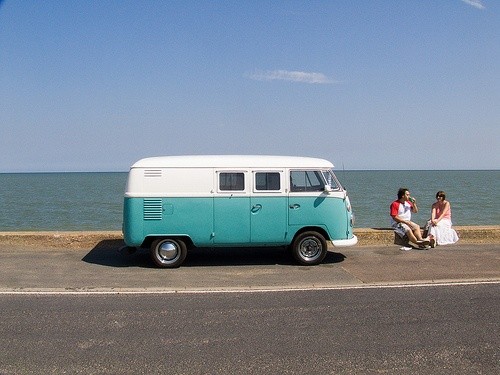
437, 194, 443, 197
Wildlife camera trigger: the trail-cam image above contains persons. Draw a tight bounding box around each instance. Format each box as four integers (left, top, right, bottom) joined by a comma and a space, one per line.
390, 188, 432, 252
423, 191, 460, 250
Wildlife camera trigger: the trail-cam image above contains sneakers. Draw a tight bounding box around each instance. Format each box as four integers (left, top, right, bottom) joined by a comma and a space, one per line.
416, 238, 431, 249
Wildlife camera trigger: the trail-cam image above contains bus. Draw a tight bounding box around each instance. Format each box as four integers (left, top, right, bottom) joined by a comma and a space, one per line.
121, 155, 359, 265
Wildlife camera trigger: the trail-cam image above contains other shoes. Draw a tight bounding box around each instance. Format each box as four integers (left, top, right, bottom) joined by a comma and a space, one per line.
430, 238, 436, 248
399, 246, 412, 251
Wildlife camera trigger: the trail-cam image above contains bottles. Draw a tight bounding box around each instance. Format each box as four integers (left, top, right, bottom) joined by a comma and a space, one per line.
409, 197, 415, 203
